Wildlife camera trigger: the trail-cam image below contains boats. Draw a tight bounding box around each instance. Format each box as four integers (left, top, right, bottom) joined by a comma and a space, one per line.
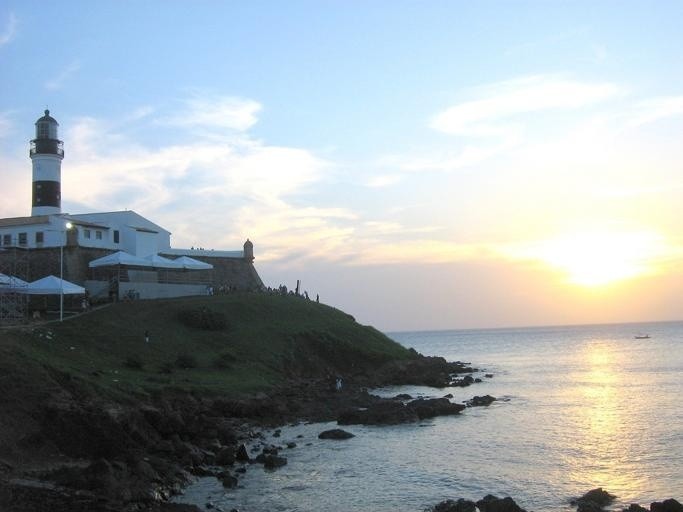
633, 334, 650, 339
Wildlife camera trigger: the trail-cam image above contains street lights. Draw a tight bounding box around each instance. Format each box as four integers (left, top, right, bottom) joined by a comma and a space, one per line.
59, 222, 71, 323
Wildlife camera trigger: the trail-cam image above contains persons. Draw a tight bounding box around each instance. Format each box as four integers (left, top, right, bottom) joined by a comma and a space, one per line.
108, 279, 136, 305
267, 284, 320, 303
335, 376, 344, 393
205, 282, 233, 297
79, 289, 90, 307
